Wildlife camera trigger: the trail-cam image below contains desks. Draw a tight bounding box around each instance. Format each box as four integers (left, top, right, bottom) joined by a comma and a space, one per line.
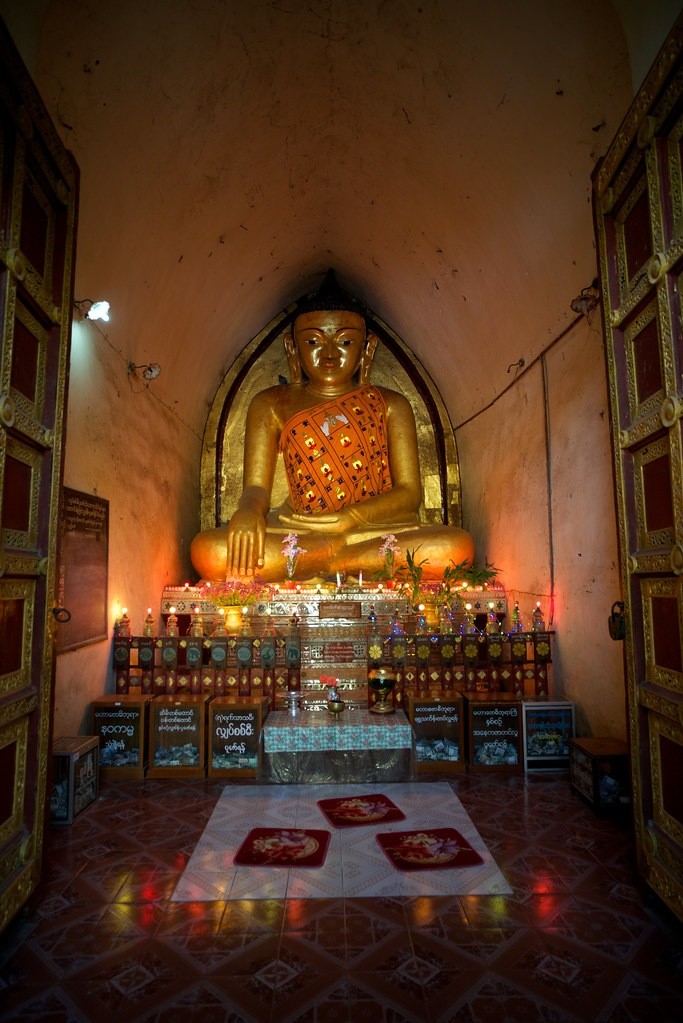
263, 708, 412, 782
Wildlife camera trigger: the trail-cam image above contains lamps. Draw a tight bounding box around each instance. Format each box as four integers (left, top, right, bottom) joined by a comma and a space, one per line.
129, 362, 161, 379
75, 299, 111, 322
570, 278, 599, 315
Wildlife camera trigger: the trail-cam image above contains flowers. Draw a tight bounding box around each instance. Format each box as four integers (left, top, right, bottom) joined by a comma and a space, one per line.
371, 534, 408, 582
407, 544, 503, 606
319, 675, 337, 686
201, 576, 274, 611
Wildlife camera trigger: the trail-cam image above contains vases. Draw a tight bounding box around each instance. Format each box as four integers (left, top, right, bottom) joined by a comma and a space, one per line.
327, 686, 340, 714
386, 580, 394, 588
219, 606, 243, 636
286, 579, 296, 588
281, 532, 307, 578
419, 603, 441, 633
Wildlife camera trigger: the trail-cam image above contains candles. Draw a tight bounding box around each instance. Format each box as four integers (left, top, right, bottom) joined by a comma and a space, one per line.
537, 607, 540, 610
516, 606, 518, 609
336, 574, 340, 588
148, 615, 152, 617
123, 614, 126, 617
396, 611, 398, 614
359, 573, 363, 586
171, 614, 174, 616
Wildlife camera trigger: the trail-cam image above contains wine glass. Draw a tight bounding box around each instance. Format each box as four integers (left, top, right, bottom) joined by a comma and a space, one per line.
368, 667, 396, 714
328, 700, 346, 722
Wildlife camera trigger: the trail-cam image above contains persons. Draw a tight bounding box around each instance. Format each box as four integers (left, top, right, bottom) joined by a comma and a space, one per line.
189, 258, 475, 575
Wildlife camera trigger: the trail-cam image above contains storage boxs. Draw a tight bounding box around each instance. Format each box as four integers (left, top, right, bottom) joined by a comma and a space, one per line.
570, 737, 630, 809
404, 690, 467, 774
463, 691, 521, 772
209, 696, 268, 778
150, 695, 212, 778
44, 736, 99, 824
90, 695, 151, 781
521, 696, 577, 772
